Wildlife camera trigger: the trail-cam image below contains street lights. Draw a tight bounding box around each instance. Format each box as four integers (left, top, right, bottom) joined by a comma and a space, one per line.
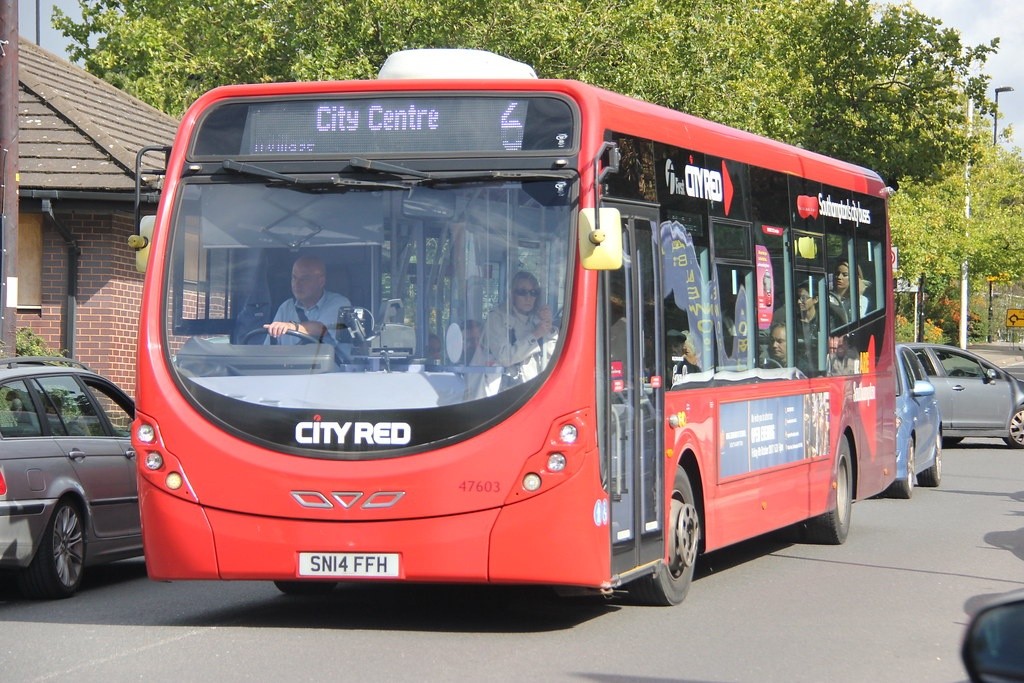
986, 86, 1015, 344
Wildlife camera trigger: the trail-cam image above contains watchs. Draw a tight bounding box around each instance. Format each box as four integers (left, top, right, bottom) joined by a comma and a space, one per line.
290, 320, 299, 332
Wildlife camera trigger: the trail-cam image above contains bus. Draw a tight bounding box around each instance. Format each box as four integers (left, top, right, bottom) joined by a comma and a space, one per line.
126, 49, 899, 607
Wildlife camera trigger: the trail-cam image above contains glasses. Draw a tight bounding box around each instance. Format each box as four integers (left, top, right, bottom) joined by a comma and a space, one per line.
795, 295, 814, 301
513, 289, 540, 296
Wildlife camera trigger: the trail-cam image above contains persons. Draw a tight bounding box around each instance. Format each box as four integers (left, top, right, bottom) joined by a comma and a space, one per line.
665, 296, 705, 386
469, 272, 560, 394
762, 259, 872, 378
258, 255, 352, 364
428, 319, 481, 368
666, 328, 702, 390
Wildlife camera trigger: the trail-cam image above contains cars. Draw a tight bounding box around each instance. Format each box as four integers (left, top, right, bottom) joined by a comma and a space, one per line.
900, 342, 1024, 449
0, 356, 144, 601
885, 342, 943, 500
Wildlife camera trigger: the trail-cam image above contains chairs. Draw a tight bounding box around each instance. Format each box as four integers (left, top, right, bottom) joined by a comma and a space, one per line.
43, 390, 85, 436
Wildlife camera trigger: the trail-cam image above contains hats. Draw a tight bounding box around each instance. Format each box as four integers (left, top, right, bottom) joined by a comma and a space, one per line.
666, 329, 687, 342
